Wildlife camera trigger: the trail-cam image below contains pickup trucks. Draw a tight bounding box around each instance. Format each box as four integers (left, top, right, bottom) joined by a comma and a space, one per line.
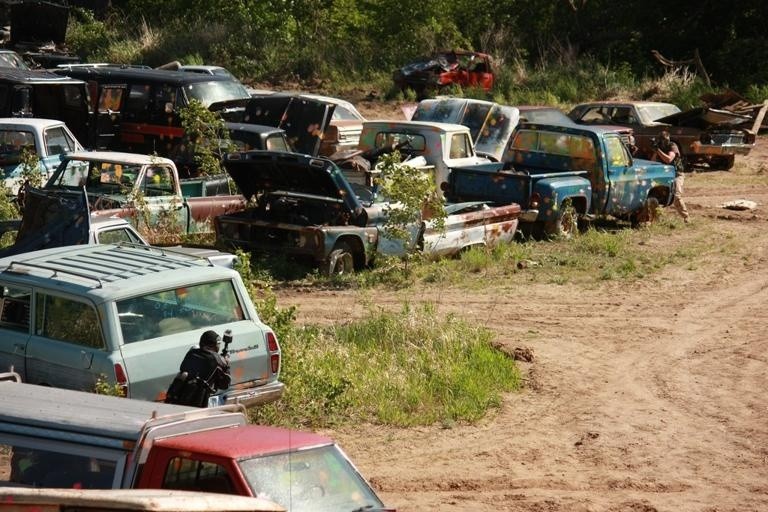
341, 121, 490, 203
214, 152, 520, 278
0, 180, 238, 327
0, 120, 88, 193
42, 153, 246, 247
448, 123, 675, 240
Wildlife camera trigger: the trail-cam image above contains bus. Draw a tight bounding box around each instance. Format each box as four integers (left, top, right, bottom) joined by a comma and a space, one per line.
54, 64, 251, 177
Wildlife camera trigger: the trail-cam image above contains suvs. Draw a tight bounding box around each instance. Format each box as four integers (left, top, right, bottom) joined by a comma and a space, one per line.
0, 50, 87, 117
0, 244, 285, 410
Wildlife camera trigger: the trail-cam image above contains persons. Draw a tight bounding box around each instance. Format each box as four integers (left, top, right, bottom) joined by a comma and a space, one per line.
162, 330, 233, 406
650, 131, 691, 223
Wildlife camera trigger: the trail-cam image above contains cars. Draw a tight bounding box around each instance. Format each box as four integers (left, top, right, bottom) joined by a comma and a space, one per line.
517, 107, 639, 156
566, 102, 755, 170
248, 95, 366, 160
393, 51, 494, 97
406, 97, 519, 162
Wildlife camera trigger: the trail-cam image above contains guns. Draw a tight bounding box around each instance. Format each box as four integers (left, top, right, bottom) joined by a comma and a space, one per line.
650, 137, 662, 150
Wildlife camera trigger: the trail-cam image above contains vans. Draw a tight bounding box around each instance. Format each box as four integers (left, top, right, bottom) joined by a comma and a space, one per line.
225, 124, 291, 154
178, 66, 245, 84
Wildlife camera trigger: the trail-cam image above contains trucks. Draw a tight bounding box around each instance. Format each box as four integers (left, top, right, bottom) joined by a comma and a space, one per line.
0, 373, 396, 511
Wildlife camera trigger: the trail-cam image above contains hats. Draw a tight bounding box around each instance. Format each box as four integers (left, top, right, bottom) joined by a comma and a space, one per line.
200, 330, 221, 346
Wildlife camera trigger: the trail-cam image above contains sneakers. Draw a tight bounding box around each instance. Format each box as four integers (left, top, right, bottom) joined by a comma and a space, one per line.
684, 216, 691, 227
